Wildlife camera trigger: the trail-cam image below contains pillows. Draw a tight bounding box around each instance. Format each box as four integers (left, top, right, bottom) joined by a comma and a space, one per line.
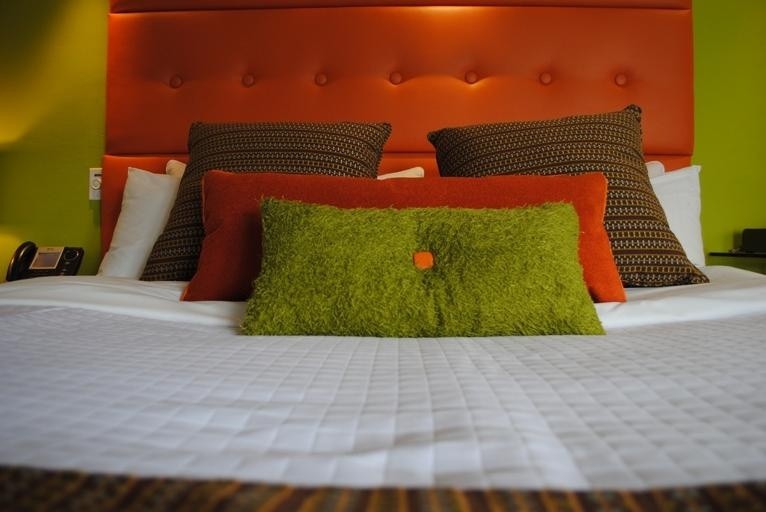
139, 119, 394, 283
426, 104, 710, 288
645, 160, 663, 178
95, 166, 426, 276
160, 156, 187, 178
180, 168, 626, 303
238, 195, 604, 337
648, 164, 706, 275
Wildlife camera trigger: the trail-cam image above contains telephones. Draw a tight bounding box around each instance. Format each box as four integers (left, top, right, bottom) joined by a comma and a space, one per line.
6, 241, 85, 281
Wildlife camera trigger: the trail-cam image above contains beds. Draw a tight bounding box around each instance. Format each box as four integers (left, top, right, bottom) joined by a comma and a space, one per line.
0, 2, 766, 509
